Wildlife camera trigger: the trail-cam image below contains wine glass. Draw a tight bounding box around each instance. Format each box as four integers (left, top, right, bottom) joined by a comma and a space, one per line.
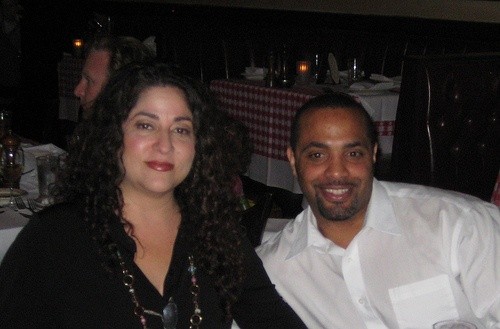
0, 145, 25, 216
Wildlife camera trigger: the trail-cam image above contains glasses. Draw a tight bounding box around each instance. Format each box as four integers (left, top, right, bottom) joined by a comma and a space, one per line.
162, 296, 178, 329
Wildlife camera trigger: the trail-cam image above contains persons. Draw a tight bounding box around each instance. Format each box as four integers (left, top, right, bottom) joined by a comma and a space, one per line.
0, 59, 308, 329
231, 91, 499, 329
74, 36, 157, 121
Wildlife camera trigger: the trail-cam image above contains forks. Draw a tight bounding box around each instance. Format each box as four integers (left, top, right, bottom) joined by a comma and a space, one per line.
13, 197, 45, 214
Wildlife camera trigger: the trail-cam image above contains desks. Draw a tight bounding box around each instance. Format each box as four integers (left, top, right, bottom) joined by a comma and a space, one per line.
209, 77, 403, 194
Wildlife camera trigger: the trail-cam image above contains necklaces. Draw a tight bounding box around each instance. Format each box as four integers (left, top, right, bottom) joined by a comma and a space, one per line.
113, 248, 203, 329
